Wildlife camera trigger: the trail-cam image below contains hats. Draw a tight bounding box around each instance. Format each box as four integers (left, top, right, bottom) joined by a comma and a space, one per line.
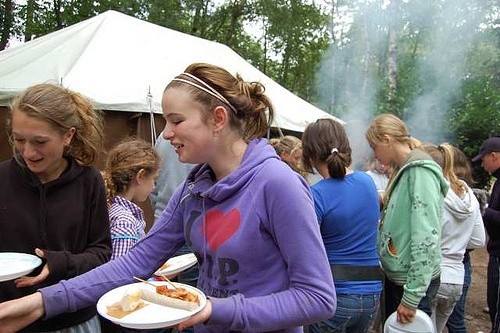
471, 137, 500, 163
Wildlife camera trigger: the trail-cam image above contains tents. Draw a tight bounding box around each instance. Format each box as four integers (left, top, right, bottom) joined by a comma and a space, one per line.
0, 9, 347, 134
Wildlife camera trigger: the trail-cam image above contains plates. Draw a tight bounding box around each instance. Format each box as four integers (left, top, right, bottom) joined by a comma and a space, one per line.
97, 281, 206, 329
0, 253, 42, 281
384, 310, 434, 333
154, 253, 198, 276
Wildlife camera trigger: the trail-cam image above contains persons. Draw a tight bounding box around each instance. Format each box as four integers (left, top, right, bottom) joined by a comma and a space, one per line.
442, 143, 475, 333
0, 63, 337, 333
478, 137, 500, 333
100, 139, 161, 333
364, 114, 450, 333
148, 129, 200, 226
270, 135, 303, 166
302, 118, 382, 333
366, 151, 393, 198
423, 145, 486, 333
0, 83, 113, 333
